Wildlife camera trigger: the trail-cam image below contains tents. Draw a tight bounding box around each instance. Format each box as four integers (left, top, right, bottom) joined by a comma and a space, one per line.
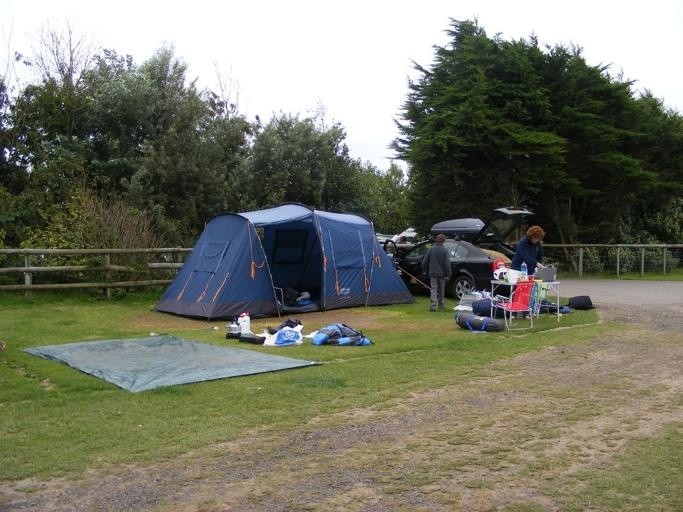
153, 202, 417, 320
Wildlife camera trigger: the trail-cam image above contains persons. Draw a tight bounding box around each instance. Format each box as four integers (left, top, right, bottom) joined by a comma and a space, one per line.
492, 258, 509, 298
398, 236, 412, 244
510, 225, 545, 321
421, 234, 452, 312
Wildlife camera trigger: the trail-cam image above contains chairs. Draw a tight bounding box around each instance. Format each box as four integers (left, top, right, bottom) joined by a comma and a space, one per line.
492, 280, 541, 332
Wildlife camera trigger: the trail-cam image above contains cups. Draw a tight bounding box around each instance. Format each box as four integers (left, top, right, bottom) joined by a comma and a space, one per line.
504, 275, 508, 282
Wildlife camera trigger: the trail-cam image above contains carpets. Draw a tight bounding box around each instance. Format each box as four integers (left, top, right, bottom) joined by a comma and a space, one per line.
16, 329, 326, 395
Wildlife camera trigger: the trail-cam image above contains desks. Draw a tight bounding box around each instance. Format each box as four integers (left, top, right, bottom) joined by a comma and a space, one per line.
487, 278, 562, 325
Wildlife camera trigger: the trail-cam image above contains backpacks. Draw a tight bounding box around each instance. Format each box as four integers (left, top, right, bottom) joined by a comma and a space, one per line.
568, 295, 594, 311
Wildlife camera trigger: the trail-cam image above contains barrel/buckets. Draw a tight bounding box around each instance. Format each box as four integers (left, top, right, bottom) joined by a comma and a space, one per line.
492, 257, 506, 271
237, 312, 250, 333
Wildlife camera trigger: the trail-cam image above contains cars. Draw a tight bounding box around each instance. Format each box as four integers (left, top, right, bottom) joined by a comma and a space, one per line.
381, 206, 536, 300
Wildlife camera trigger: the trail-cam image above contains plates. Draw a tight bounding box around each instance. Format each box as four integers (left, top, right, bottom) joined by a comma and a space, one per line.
534, 280, 543, 282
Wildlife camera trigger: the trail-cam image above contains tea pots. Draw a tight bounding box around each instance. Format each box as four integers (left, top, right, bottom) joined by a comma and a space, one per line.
228, 318, 239, 332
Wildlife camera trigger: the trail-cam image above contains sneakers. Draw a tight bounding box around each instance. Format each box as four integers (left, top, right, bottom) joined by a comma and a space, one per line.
429, 306, 447, 312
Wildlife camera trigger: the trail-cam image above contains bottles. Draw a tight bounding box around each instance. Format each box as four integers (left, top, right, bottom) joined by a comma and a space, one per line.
239, 313, 251, 334
520, 261, 527, 279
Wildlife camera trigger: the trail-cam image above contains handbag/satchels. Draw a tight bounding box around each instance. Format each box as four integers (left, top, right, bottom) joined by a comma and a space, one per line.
534, 265, 558, 283
